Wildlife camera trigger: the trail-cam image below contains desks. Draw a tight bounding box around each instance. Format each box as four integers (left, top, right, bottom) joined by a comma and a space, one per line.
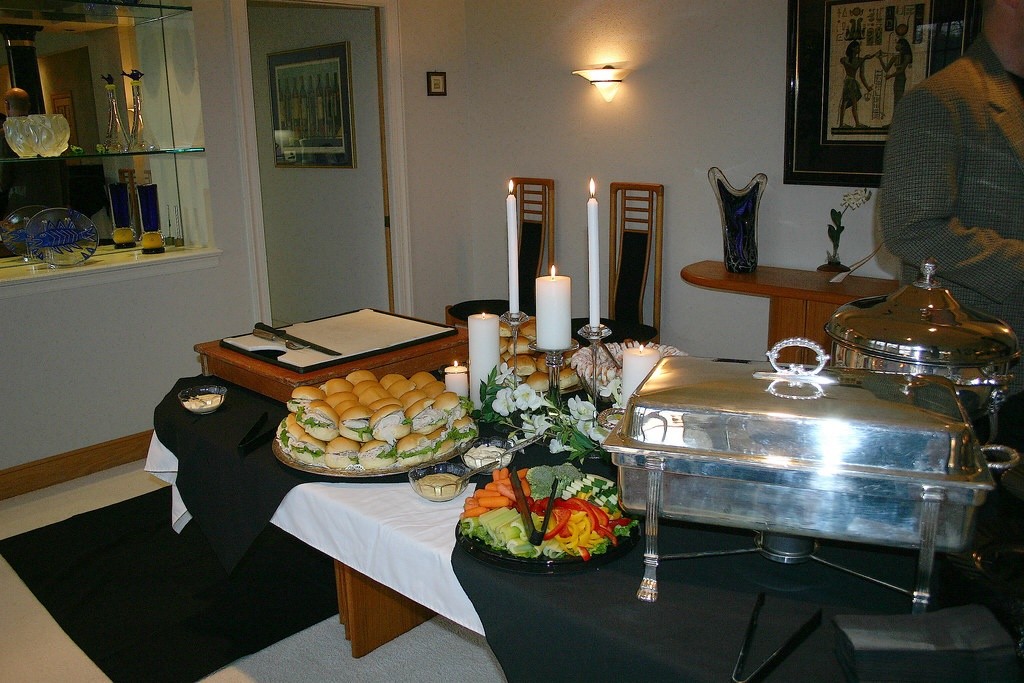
682, 261, 902, 364
143, 368, 1024, 683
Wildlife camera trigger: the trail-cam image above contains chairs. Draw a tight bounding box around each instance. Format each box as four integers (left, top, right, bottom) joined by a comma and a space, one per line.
445, 176, 555, 330
571, 182, 664, 347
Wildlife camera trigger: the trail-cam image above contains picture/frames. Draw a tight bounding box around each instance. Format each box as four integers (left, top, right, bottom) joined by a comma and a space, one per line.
51, 93, 78, 154
266, 40, 358, 171
783, 0, 984, 189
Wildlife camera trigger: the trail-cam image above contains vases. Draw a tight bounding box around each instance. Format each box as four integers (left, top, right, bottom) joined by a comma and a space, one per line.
815, 261, 851, 274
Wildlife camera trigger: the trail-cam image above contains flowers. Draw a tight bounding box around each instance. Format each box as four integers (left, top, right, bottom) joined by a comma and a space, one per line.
826, 188, 872, 262
475, 361, 623, 470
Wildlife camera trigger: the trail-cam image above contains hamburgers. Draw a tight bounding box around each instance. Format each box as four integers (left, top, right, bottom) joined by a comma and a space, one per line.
275, 370, 479, 470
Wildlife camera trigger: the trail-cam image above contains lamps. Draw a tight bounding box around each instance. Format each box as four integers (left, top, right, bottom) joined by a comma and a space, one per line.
570, 65, 633, 104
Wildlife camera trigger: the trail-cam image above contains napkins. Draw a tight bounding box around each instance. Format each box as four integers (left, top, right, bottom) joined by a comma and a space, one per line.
830, 602, 1018, 683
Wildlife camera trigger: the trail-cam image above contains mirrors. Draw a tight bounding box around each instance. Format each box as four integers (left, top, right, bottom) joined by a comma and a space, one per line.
0, 0, 183, 271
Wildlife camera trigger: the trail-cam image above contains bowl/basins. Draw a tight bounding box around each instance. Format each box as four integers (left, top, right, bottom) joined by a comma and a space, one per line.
178, 384, 227, 415
459, 436, 516, 475
410, 462, 470, 502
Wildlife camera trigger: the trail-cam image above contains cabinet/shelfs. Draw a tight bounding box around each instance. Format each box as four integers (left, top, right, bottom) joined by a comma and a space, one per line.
0, 0, 223, 300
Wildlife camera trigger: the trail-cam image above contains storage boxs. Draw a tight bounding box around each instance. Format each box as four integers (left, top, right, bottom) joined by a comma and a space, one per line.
597, 354, 995, 554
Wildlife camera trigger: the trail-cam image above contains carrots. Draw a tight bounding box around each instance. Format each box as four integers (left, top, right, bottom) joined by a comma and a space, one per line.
459, 468, 531, 519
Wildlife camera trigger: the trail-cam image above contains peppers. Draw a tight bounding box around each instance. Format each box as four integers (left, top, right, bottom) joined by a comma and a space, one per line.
513, 494, 632, 561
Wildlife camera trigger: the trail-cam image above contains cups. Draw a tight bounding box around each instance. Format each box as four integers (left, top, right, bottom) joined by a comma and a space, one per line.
27, 114, 69, 157
3, 115, 38, 157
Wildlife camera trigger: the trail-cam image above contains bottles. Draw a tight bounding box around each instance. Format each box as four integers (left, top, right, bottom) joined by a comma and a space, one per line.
129, 81, 160, 152
104, 84, 129, 153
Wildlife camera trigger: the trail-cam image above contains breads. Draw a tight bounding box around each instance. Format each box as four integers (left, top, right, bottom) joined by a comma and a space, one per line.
497, 318, 582, 393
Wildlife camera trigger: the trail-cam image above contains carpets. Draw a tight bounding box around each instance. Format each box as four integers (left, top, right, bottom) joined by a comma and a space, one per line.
0, 485, 339, 683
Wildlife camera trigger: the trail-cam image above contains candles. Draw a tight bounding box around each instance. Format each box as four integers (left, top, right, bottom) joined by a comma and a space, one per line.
585, 177, 602, 329
467, 312, 502, 411
444, 360, 470, 399
621, 344, 661, 408
506, 178, 521, 313
535, 265, 571, 350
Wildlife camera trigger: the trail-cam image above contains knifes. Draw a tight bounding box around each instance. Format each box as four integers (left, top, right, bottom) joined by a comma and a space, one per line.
254, 322, 342, 356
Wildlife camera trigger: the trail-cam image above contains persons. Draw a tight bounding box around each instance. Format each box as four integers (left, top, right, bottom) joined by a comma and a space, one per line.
879, 0, 1024, 452
0, 88, 70, 221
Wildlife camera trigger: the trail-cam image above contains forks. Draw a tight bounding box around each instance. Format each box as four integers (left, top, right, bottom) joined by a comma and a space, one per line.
252, 329, 312, 351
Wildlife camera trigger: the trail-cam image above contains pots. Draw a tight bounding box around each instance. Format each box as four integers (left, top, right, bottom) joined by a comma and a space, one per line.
826, 259, 1022, 424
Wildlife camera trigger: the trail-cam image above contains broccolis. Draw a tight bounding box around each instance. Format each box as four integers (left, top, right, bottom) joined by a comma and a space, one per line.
527, 463, 585, 501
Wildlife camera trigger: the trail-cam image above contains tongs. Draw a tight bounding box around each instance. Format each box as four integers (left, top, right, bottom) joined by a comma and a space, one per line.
731, 592, 826, 683
237, 412, 286, 458
509, 466, 559, 545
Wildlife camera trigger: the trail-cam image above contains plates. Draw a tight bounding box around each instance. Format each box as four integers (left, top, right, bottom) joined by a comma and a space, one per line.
1, 205, 47, 258
26, 208, 100, 267
456, 507, 642, 575
271, 427, 478, 476
571, 341, 689, 379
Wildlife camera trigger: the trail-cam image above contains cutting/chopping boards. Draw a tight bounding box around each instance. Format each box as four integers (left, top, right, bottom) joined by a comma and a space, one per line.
219, 308, 459, 374
